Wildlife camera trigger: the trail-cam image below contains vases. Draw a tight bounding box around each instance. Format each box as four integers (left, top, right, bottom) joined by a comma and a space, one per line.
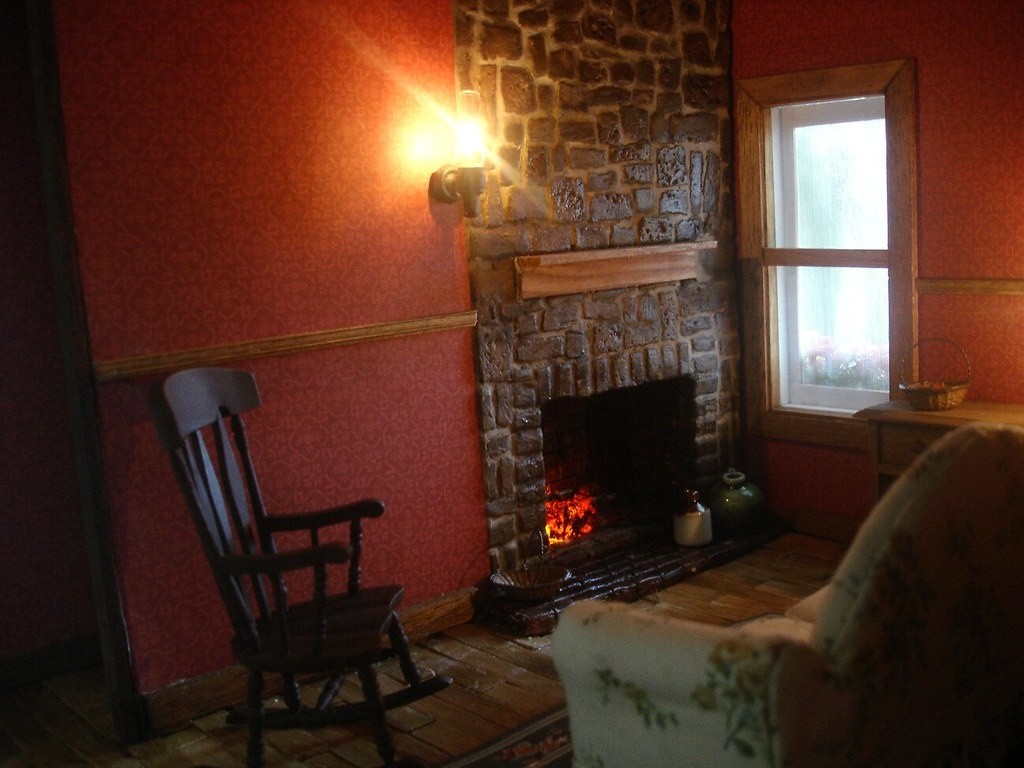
672, 469, 764, 546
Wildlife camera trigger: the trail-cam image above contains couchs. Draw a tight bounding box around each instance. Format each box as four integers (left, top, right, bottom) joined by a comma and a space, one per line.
551, 422, 1024, 768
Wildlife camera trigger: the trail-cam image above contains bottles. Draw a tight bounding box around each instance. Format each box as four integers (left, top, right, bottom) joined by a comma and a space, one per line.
672, 487, 712, 545
711, 469, 764, 533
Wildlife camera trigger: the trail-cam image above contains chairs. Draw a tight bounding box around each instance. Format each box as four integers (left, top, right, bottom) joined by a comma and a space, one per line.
153, 367, 453, 768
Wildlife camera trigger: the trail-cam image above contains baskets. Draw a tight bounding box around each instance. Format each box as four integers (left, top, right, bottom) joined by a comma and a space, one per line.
897, 338, 973, 410
490, 526, 572, 601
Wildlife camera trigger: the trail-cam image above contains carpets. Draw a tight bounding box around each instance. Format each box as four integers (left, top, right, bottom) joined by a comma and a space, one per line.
440, 700, 573, 768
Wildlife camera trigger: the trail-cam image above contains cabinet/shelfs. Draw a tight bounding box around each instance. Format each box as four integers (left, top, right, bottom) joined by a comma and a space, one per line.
851, 399, 1024, 506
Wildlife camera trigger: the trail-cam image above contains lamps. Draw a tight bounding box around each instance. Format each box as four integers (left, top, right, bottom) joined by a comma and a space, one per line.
430, 91, 492, 219
